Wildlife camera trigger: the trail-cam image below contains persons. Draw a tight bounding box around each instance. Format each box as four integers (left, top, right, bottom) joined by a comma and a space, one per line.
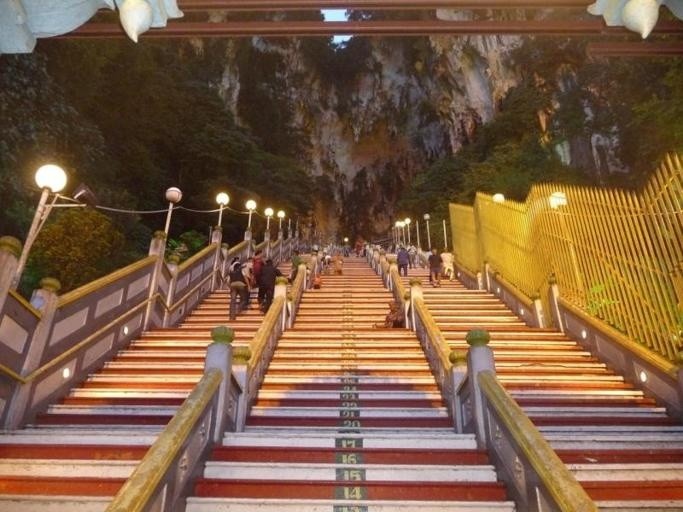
392, 240, 456, 288
327, 249, 344, 276
227, 243, 334, 321
